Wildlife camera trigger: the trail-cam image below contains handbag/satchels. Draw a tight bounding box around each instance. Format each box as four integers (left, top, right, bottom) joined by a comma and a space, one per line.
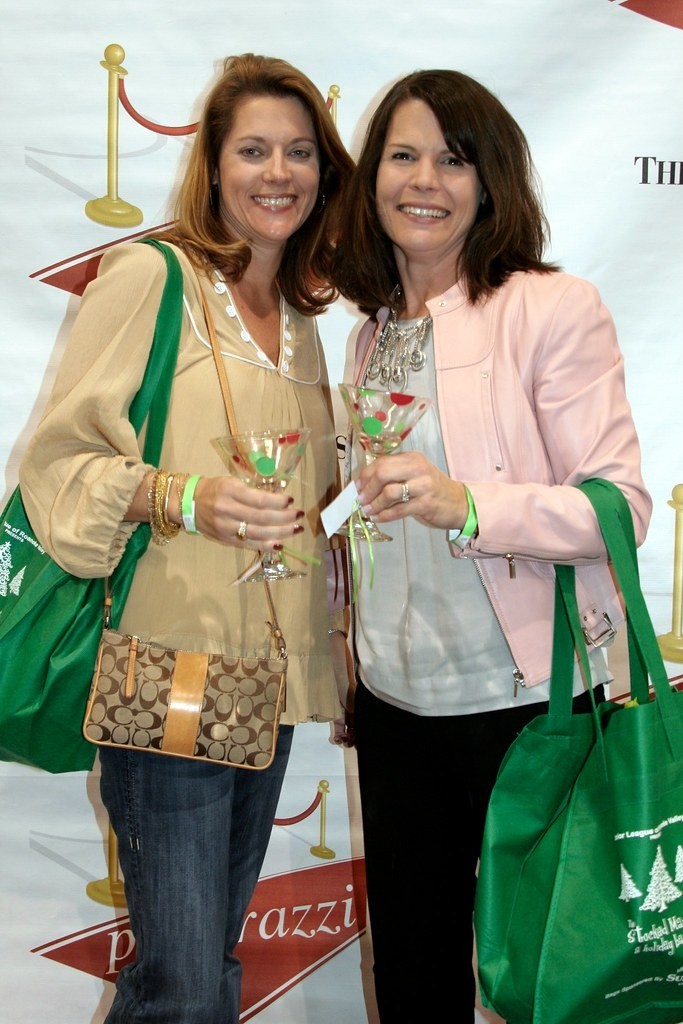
83, 628, 288, 770
0, 238, 184, 774
473, 477, 683, 1024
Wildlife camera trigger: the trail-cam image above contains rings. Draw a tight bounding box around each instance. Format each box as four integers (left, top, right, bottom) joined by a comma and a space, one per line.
401, 482, 410, 504
236, 520, 247, 542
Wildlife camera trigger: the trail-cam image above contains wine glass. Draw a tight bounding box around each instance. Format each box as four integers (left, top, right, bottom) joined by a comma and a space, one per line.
208, 427, 312, 586
332, 382, 435, 542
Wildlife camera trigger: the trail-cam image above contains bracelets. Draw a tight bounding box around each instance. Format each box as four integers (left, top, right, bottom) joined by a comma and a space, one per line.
146, 468, 203, 546
448, 484, 478, 550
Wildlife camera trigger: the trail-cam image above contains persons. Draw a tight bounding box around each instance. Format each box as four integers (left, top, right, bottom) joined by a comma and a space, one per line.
20, 53, 359, 1024
326, 69, 652, 1024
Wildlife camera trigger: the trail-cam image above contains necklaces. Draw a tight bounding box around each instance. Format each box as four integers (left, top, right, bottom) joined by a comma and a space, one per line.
366, 288, 432, 393
